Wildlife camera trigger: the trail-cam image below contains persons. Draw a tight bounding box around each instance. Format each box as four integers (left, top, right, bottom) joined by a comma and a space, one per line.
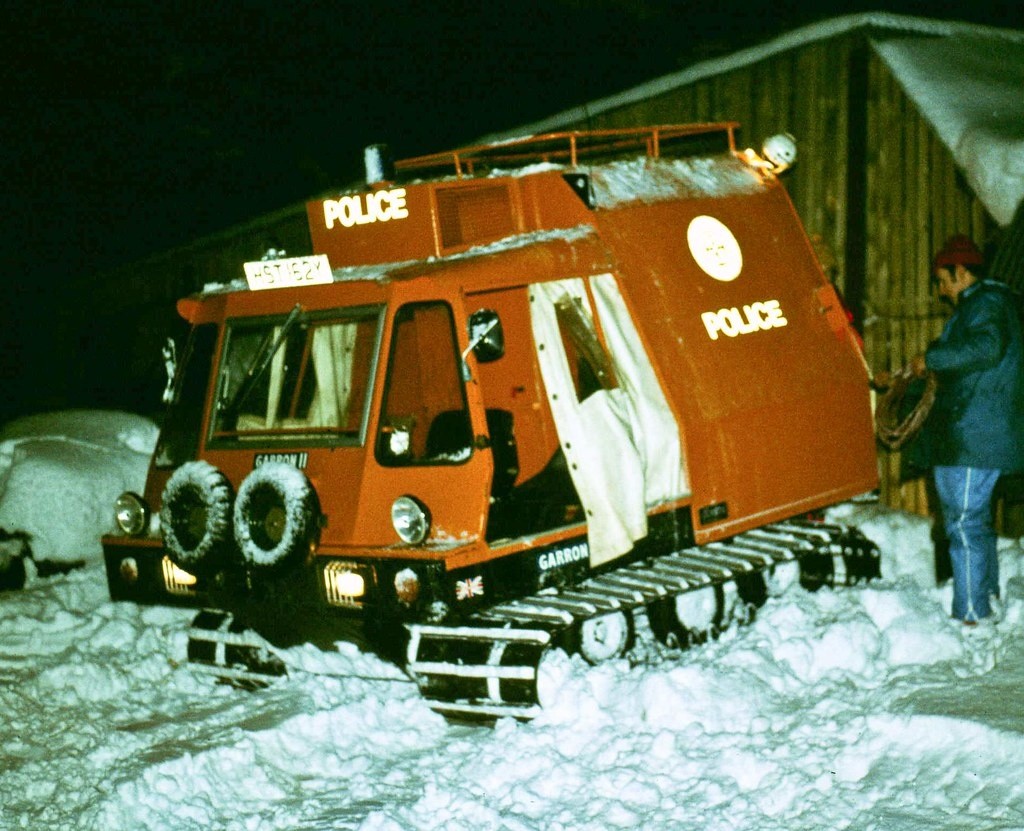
875, 235, 1024, 628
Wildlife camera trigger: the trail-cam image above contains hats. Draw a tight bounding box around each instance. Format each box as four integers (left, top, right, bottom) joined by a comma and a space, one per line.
937, 235, 985, 268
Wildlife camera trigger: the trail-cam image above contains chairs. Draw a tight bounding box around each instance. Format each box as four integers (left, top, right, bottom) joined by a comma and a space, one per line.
424, 409, 517, 508
